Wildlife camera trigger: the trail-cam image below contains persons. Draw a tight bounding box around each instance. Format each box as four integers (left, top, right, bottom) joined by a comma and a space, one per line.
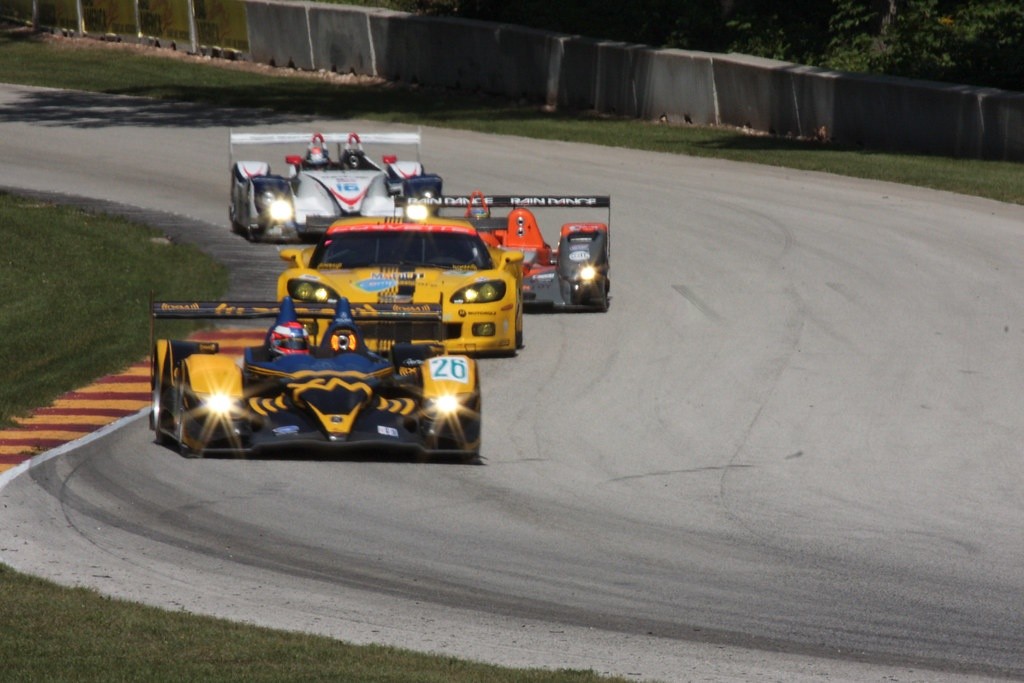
472, 209, 488, 219
268, 321, 310, 361
310, 147, 324, 162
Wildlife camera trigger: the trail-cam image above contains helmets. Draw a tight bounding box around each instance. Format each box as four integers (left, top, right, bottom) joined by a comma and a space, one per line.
310, 148, 323, 161
265, 320, 312, 358
418, 186, 440, 215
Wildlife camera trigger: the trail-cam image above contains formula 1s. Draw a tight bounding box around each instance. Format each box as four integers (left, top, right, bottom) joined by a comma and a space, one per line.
408, 193, 617, 312
227, 124, 445, 241
147, 269, 486, 464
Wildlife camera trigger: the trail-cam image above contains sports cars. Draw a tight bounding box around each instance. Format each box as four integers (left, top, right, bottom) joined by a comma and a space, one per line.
275, 206, 531, 357
